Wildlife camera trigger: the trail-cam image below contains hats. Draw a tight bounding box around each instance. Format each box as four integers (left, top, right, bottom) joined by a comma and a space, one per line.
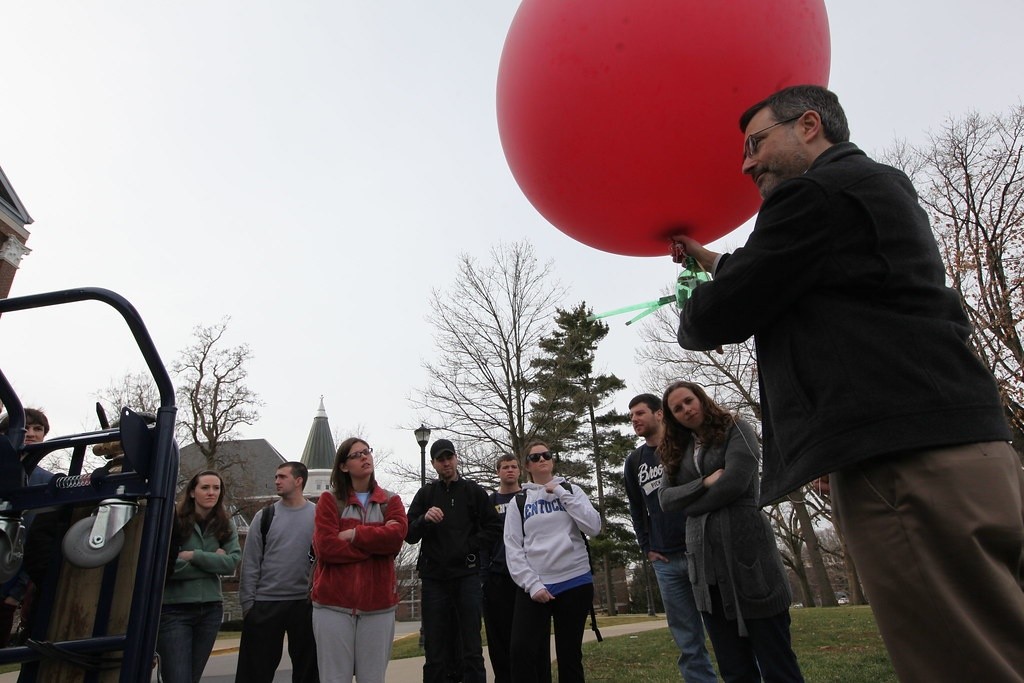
430, 439, 455, 459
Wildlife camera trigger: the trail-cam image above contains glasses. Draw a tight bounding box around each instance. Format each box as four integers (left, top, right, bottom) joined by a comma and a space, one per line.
744, 111, 809, 161
528, 450, 552, 463
344, 448, 373, 459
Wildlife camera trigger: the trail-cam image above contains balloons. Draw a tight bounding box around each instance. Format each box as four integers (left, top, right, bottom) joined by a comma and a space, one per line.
495, 0, 831, 256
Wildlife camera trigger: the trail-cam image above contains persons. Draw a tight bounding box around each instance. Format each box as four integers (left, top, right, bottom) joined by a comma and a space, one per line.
23, 408, 56, 486
480, 442, 602, 683
623, 381, 806, 683
157, 470, 242, 683
235, 462, 319, 683
628, 592, 635, 615
668, 85, 1024, 683
404, 439, 487, 683
311, 437, 409, 683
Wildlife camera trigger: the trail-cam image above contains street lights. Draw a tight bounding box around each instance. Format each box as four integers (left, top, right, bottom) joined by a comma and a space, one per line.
412, 423, 433, 493
